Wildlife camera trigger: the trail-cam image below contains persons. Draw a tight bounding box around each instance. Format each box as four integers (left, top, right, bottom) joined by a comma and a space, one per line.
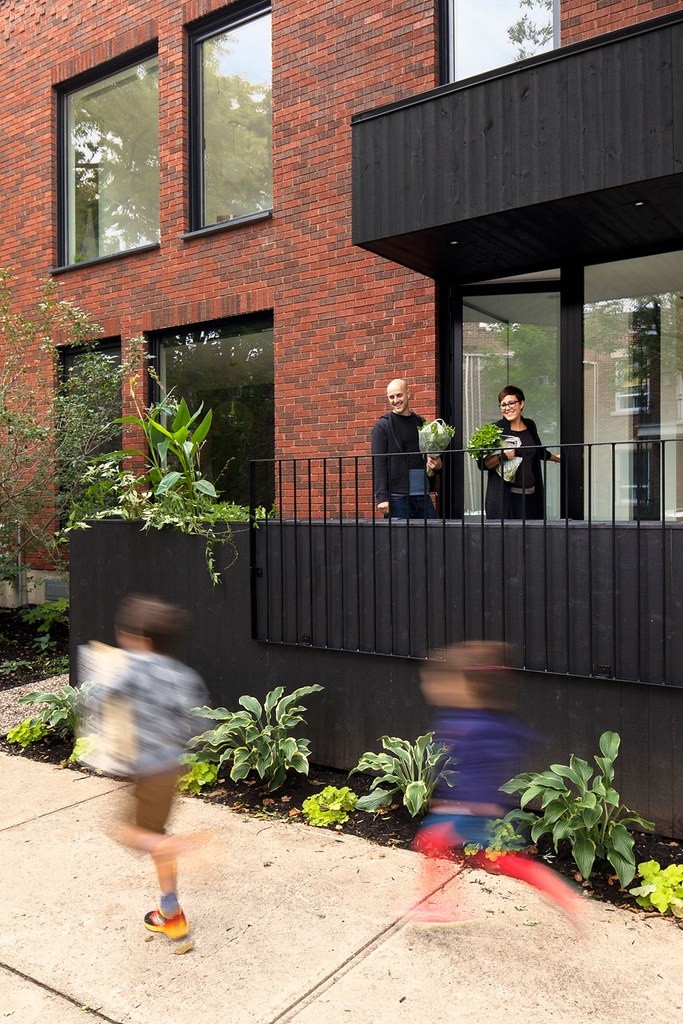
70, 592, 226, 940
371, 379, 447, 518
475, 385, 561, 521
395, 642, 593, 948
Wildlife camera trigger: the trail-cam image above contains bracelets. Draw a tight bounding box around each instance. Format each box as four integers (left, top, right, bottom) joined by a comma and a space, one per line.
497, 452, 509, 463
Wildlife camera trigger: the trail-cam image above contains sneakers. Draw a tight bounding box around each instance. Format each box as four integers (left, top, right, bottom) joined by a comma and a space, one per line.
144, 909, 188, 939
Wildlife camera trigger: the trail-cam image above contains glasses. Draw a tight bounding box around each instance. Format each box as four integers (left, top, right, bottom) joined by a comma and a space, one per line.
498, 400, 520, 410
